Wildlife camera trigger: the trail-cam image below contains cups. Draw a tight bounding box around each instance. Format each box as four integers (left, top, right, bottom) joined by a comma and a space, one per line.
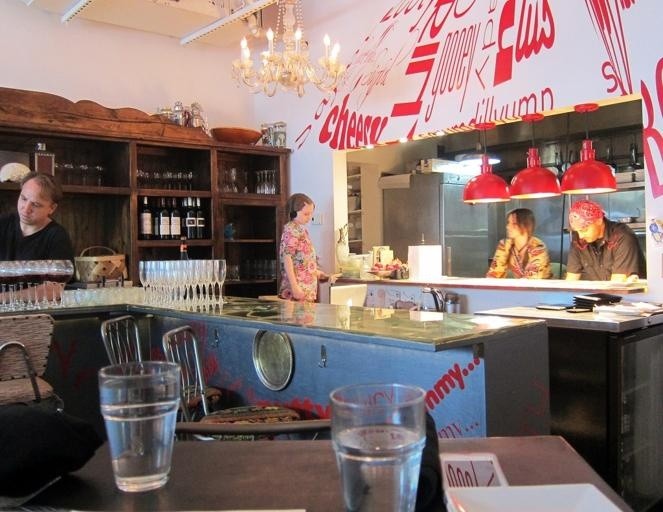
61, 288, 144, 307
98, 359, 181, 494
329, 383, 427, 512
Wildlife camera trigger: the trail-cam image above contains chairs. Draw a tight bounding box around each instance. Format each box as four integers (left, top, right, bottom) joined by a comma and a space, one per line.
1, 314, 65, 479
161, 326, 301, 443
97, 315, 221, 440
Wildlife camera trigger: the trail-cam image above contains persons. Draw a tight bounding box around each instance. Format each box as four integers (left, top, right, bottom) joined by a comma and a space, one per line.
1, 168, 74, 304
276, 192, 329, 302
565, 199, 647, 281
485, 207, 552, 280
279, 299, 319, 328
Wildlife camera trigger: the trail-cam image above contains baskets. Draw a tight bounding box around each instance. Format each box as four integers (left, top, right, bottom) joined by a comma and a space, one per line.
74, 246, 129, 283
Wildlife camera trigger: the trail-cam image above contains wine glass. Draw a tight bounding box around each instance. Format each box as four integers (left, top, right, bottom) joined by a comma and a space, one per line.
136, 169, 193, 191
0, 259, 74, 313
254, 169, 277, 195
138, 259, 226, 309
53, 161, 103, 187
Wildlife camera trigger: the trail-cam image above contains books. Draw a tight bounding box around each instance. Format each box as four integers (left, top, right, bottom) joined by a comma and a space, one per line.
536, 291, 663, 318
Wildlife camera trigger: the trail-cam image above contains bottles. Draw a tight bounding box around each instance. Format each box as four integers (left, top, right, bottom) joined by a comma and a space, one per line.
261, 124, 274, 146
178, 236, 189, 259
444, 292, 460, 314
358, 255, 371, 280
139, 196, 205, 239
273, 121, 287, 146
244, 257, 275, 280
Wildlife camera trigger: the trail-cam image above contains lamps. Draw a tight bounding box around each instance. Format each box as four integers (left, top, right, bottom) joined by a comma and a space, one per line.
462, 121, 511, 203
561, 103, 617, 195
510, 113, 561, 202
461, 129, 501, 165
230, 1, 343, 99
553, 132, 644, 183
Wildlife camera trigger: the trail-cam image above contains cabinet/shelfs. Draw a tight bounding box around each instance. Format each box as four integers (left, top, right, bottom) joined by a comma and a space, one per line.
0, 88, 292, 299
346, 166, 362, 252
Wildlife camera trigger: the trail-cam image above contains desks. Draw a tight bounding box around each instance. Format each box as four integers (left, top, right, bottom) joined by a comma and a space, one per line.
10, 435, 638, 512
